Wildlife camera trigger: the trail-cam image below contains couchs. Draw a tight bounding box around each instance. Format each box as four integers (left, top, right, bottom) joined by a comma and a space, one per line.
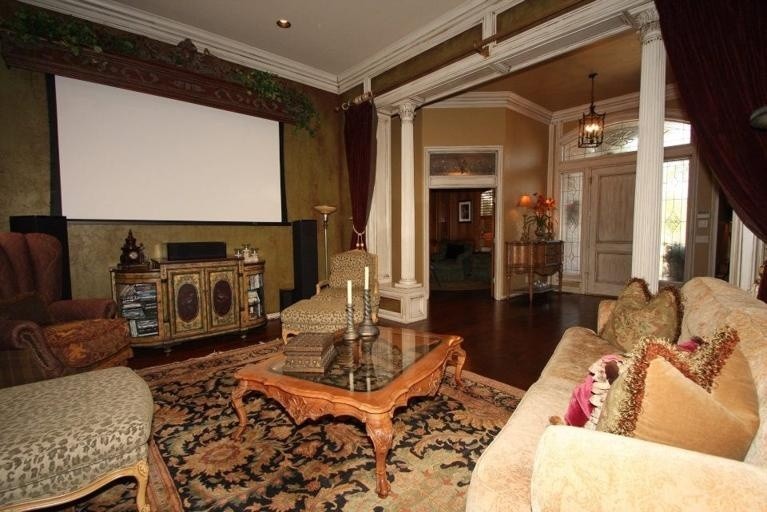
463, 276, 767, 511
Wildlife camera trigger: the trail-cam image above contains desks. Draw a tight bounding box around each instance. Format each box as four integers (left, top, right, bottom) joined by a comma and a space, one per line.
507, 239, 565, 304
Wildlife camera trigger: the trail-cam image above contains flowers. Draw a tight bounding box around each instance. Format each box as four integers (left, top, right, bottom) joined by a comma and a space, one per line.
531, 192, 552, 215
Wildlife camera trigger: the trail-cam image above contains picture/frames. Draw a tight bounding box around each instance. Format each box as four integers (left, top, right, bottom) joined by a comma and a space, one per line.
456, 199, 473, 223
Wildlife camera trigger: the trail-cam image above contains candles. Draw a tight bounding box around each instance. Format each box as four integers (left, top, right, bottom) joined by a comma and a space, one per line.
364, 266, 369, 290
346, 278, 352, 307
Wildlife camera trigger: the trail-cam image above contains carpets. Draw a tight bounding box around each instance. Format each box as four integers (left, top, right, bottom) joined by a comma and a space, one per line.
41, 335, 528, 512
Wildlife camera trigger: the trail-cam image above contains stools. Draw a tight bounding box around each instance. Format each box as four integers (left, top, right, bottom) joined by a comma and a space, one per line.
0, 365, 154, 511
279, 300, 364, 346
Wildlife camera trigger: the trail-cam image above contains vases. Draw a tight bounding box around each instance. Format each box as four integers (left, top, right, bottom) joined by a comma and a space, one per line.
535, 215, 547, 242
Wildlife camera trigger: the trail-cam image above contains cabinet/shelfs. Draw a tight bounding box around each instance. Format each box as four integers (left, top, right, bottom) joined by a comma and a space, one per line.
162, 259, 242, 352
242, 259, 266, 329
110, 268, 161, 347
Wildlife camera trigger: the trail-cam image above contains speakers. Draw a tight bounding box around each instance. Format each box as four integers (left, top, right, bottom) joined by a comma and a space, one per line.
11, 215, 73, 301
293, 219, 319, 301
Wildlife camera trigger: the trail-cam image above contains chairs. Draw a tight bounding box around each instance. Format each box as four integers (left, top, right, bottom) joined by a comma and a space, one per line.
280, 248, 380, 322
0, 231, 135, 388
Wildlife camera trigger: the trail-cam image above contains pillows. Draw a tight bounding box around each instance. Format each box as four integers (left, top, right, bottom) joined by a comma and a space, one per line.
563, 334, 703, 430
594, 324, 760, 462
598, 277, 684, 356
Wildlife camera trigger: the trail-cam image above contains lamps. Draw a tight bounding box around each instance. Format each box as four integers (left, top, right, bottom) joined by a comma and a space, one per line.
516, 193, 531, 243
576, 72, 605, 148
545, 199, 555, 239
314, 205, 337, 282
